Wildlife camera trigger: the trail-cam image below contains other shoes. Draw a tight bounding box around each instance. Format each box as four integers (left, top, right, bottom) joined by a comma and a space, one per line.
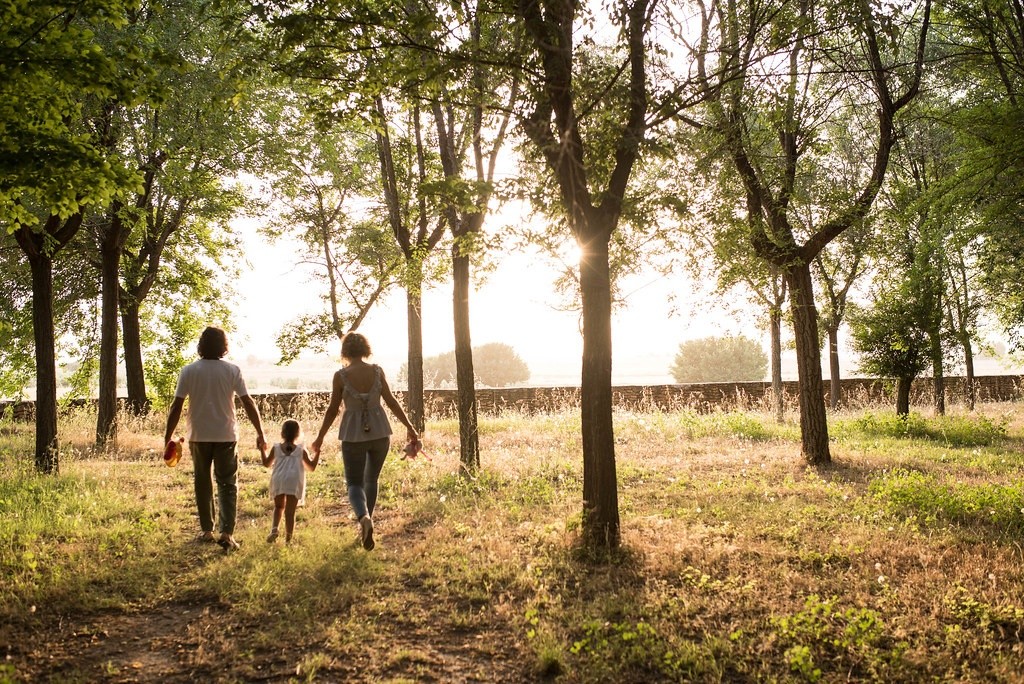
199, 531, 216, 543
267, 533, 278, 543
218, 538, 240, 551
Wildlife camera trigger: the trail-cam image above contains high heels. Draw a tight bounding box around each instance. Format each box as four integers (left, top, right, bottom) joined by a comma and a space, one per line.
361, 520, 375, 551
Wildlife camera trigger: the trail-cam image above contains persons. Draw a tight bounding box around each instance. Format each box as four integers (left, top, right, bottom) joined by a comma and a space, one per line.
165, 327, 268, 550
312, 333, 418, 550
259, 420, 320, 544
